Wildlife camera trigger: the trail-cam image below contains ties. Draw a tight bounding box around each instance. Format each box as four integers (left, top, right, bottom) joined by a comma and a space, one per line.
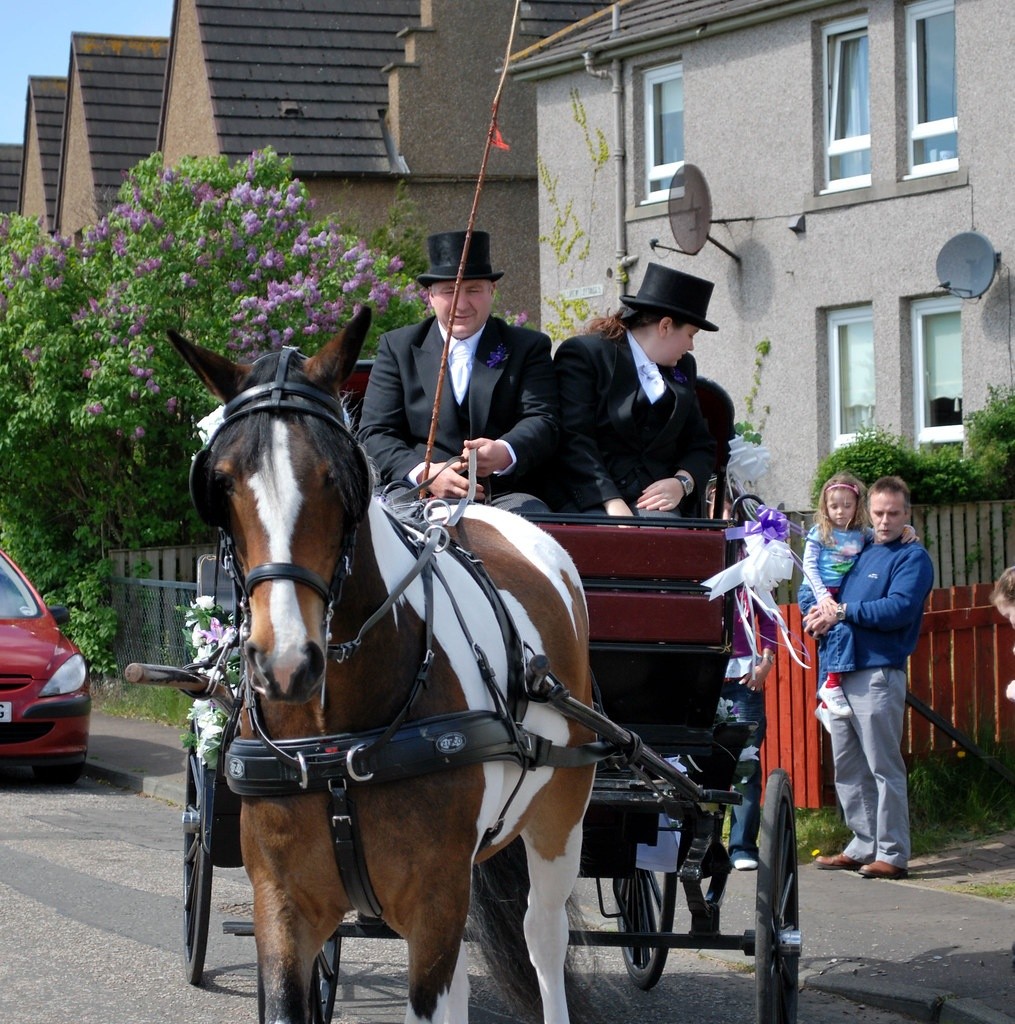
642, 364, 665, 404
449, 341, 473, 405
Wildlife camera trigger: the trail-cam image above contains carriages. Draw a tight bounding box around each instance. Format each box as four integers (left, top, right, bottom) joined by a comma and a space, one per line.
125, 304, 803, 1024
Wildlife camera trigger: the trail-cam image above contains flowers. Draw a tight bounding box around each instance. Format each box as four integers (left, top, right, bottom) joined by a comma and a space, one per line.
176, 593, 242, 770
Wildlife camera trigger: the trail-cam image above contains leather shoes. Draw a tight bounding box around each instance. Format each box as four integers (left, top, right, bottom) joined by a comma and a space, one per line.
857, 860, 908, 880
814, 853, 863, 870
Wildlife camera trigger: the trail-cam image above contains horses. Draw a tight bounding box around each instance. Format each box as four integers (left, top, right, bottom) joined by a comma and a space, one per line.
167, 308, 627, 1024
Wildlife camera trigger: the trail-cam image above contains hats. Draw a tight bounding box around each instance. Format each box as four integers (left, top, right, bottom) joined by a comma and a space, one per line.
619, 262, 719, 332
416, 229, 505, 289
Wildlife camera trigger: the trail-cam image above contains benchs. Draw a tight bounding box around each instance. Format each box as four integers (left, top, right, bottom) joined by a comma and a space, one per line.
521, 511, 736, 776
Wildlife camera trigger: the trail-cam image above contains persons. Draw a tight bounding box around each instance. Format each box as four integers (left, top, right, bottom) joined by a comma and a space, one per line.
989, 563, 1015, 703
705, 473, 777, 871
353, 231, 562, 513
798, 475, 935, 877
553, 262, 720, 528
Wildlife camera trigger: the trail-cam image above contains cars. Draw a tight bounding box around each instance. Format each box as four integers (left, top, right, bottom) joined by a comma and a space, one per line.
0, 551, 92, 789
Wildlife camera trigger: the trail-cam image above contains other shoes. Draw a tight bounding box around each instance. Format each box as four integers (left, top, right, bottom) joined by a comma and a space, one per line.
819, 680, 853, 718
815, 702, 831, 735
734, 859, 759, 871
